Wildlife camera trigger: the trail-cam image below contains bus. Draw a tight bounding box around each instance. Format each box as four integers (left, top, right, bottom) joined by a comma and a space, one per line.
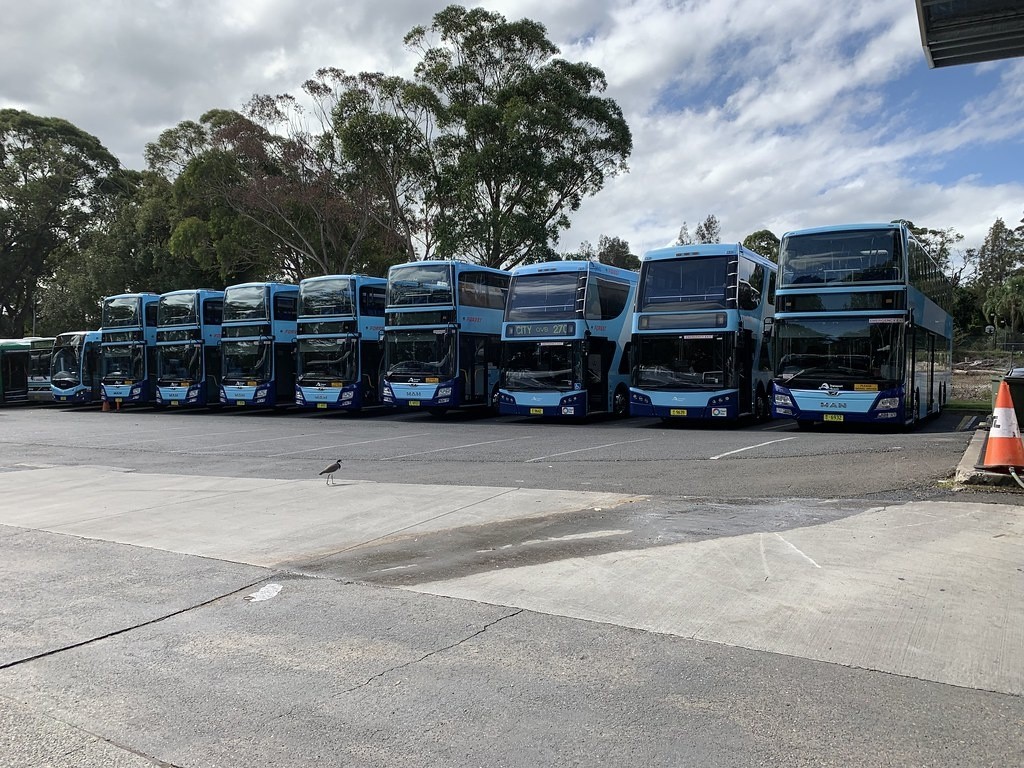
28, 337, 55, 404
223, 282, 299, 412
1, 336, 42, 406
770, 221, 954, 428
153, 288, 225, 410
632, 245, 778, 423
49, 327, 104, 410
98, 292, 159, 408
386, 261, 513, 416
500, 261, 640, 420
298, 273, 388, 413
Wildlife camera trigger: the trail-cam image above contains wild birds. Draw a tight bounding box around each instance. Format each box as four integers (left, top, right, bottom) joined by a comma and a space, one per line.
319, 459, 344, 485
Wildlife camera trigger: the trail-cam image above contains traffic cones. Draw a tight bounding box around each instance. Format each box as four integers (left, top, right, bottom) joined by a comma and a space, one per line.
101, 400, 111, 411
974, 380, 1023, 471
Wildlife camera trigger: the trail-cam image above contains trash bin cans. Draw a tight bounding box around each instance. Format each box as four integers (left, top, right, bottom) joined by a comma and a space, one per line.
991, 375, 1024, 432
